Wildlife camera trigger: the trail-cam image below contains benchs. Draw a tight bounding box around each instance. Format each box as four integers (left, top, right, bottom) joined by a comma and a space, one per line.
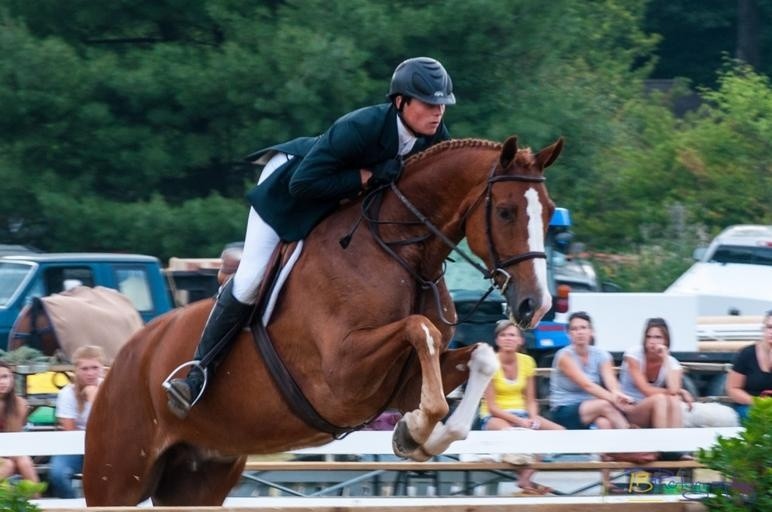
375, 361, 755, 498
22, 398, 55, 484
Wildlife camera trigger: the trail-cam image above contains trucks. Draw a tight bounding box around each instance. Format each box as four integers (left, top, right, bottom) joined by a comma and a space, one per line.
667, 224, 772, 345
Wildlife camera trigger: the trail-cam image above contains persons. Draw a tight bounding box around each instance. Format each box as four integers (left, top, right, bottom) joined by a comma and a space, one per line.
723, 309, 772, 427
0, 345, 104, 498
481, 316, 566, 496
549, 313, 694, 486
170, 57, 456, 419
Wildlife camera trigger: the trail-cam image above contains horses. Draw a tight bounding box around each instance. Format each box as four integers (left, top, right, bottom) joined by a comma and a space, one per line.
8, 283, 144, 356
82, 135, 566, 507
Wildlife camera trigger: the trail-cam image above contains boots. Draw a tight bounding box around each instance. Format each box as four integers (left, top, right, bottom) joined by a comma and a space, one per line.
168, 277, 257, 418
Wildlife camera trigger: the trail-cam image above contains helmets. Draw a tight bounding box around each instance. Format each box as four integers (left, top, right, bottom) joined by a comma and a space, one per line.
389, 56, 456, 105
